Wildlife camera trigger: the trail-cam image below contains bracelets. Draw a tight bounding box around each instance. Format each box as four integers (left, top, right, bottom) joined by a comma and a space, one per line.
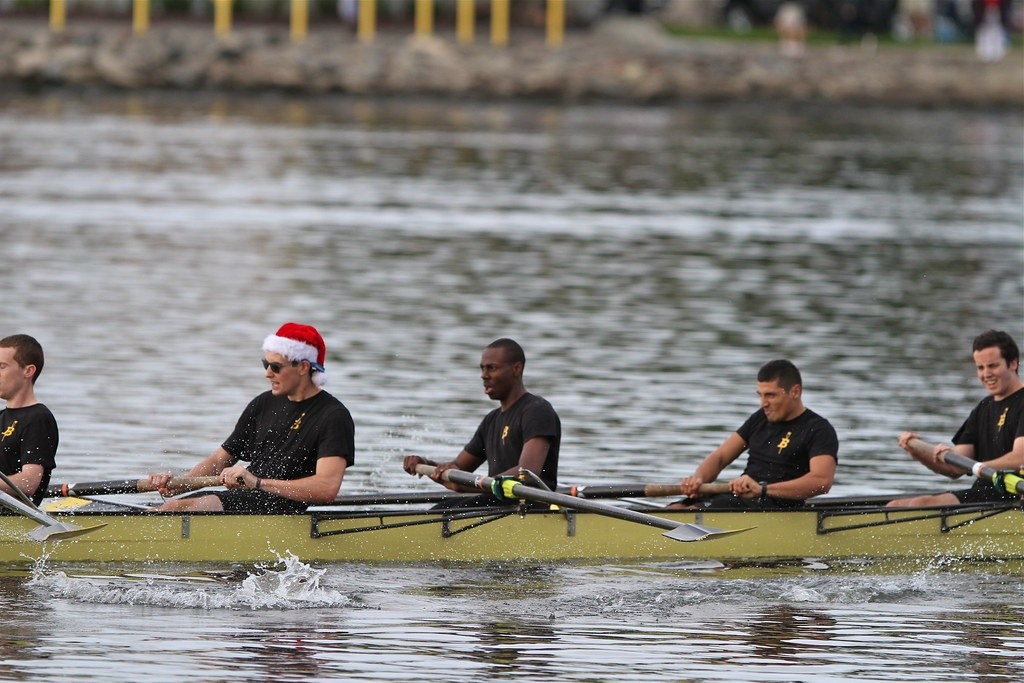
759, 481, 768, 500
256, 478, 261, 490
966, 470, 973, 477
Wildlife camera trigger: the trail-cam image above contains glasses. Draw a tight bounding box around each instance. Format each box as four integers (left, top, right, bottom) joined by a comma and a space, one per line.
262, 359, 297, 373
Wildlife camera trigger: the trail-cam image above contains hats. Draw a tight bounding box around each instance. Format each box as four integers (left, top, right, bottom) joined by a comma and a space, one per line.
263, 322, 327, 370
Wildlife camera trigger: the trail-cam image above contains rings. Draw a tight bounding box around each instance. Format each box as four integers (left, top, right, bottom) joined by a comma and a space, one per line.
684, 484, 689, 485
404, 466, 406, 467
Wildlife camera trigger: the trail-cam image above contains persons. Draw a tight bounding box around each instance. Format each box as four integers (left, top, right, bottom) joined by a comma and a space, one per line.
664, 360, 839, 509
883, 330, 1024, 508
149, 322, 355, 514
403, 338, 562, 510
0, 334, 59, 516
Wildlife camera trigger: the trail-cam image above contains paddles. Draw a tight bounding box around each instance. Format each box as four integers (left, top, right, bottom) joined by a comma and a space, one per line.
908, 438, 1023, 494
0, 492, 108, 544
42, 476, 243, 498
415, 463, 759, 543
317, 483, 731, 506
